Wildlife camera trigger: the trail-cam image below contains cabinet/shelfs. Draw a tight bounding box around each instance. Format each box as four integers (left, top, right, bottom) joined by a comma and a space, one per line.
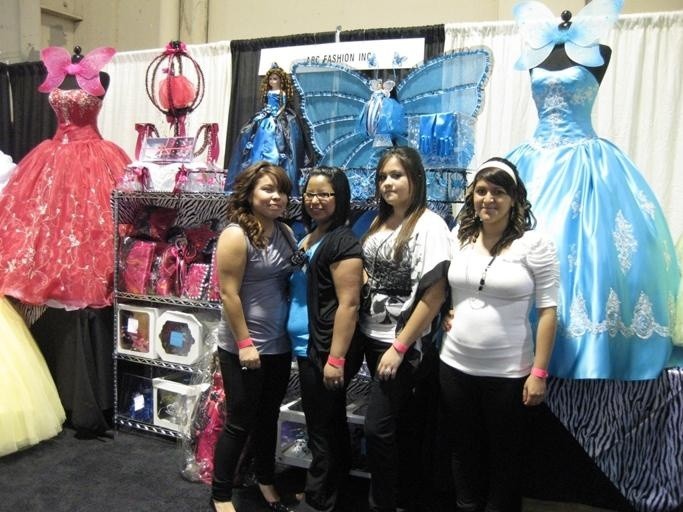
110, 191, 446, 482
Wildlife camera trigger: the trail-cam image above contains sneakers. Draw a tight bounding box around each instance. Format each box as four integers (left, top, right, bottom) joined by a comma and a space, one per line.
254, 484, 287, 511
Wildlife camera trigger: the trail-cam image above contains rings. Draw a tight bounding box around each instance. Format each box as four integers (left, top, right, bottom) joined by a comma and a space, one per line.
333, 381, 339, 385
241, 366, 248, 370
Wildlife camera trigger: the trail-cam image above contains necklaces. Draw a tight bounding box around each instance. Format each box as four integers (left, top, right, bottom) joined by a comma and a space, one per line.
474, 250, 497, 300
360, 229, 393, 300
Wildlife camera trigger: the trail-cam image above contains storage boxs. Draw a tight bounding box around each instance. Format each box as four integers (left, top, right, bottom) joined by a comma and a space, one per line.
114, 303, 218, 365
274, 401, 384, 480
148, 374, 211, 435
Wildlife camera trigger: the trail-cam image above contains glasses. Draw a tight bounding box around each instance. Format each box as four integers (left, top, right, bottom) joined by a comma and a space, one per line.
302, 192, 336, 199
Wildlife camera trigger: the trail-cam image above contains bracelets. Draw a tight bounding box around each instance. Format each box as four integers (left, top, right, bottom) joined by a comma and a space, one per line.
391, 340, 410, 355
235, 337, 254, 348
532, 366, 550, 379
326, 354, 346, 367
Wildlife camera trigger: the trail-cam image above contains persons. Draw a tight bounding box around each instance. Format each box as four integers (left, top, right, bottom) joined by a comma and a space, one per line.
285, 162, 365, 509
223, 62, 320, 195
207, 158, 301, 512
500, 1, 682, 382
356, 145, 454, 512
0, 43, 138, 315
435, 155, 562, 511
